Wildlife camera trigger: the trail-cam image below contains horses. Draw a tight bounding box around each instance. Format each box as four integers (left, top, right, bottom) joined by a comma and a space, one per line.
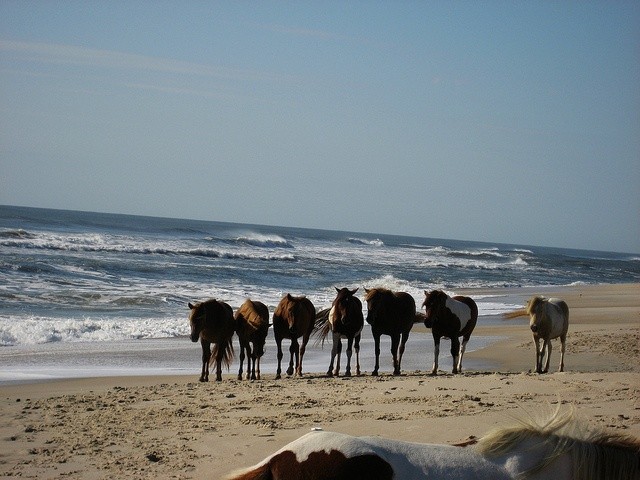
229, 396, 640, 480
422, 289, 478, 376
364, 287, 416, 377
187, 301, 235, 382
273, 292, 316, 381
235, 298, 274, 381
311, 286, 364, 378
527, 296, 569, 374
